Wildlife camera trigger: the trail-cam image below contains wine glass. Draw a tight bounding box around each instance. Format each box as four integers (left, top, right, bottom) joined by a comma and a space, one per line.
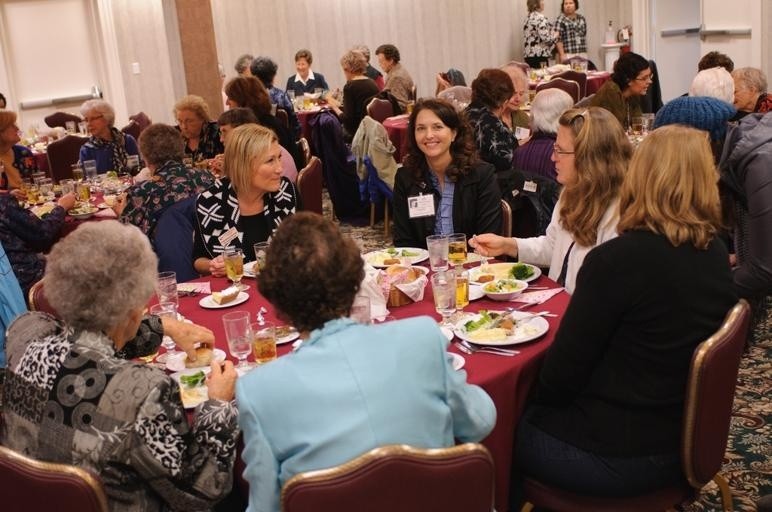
427, 233, 470, 329
350, 295, 372, 326
407, 99, 416, 124
150, 242, 277, 376
287, 88, 322, 109
21, 155, 139, 207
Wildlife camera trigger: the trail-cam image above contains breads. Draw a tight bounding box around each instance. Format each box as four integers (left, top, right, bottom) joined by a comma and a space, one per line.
185, 347, 213, 368
387, 264, 416, 284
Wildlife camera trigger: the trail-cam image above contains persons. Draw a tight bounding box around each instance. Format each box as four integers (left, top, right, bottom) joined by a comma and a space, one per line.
511, 124, 741, 500
1, 93, 300, 295
392, 45, 772, 296
649, 97, 772, 313
554, 0, 588, 69
234, 45, 417, 169
0, 220, 237, 507
227, 211, 498, 512
524, 0, 559, 68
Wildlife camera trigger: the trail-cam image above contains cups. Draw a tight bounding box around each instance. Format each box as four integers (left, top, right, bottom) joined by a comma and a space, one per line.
570, 59, 588, 74
632, 113, 655, 135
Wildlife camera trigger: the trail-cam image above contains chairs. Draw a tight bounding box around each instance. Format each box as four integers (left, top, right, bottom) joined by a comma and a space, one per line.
0, 447, 108, 511
520, 299, 752, 511
280, 442, 495, 512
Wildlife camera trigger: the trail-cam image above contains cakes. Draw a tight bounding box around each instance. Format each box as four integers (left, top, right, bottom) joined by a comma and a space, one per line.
212, 286, 240, 304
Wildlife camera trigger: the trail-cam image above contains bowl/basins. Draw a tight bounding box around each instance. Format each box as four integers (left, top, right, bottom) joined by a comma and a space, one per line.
482, 279, 528, 301
68, 208, 99, 219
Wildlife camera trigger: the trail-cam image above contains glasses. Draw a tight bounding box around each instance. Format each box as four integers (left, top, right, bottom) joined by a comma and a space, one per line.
635, 73, 653, 80
554, 143, 574, 154
85, 115, 103, 122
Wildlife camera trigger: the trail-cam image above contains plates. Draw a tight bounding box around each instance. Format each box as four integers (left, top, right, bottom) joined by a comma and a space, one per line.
462, 263, 542, 287
256, 326, 299, 344
447, 352, 465, 371
168, 366, 213, 409
29, 206, 43, 219
441, 328, 454, 342
468, 284, 487, 302
364, 247, 429, 267
455, 310, 549, 345
409, 265, 429, 278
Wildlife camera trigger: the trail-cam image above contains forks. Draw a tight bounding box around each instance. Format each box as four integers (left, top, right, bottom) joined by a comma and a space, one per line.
454, 342, 515, 356
461, 340, 520, 354
